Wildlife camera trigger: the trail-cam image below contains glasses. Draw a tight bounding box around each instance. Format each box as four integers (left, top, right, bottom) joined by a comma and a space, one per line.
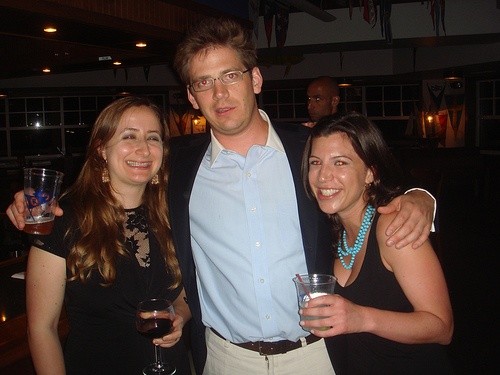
190, 68, 253, 93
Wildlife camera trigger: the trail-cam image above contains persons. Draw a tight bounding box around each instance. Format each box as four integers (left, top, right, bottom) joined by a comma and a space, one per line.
305, 76, 342, 123
24, 97, 192, 375
298, 109, 454, 375
7, 15, 437, 375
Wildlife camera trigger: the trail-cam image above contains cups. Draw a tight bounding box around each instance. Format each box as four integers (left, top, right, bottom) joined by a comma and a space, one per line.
22, 167, 64, 234
292, 273, 336, 331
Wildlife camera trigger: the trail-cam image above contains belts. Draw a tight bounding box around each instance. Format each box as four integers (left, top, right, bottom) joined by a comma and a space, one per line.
210, 328, 323, 356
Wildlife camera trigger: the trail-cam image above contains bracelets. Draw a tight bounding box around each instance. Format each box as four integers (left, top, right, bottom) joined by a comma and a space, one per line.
183, 296, 188, 304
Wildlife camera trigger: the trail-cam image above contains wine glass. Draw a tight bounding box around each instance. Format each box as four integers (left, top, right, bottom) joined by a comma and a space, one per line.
136, 298, 177, 375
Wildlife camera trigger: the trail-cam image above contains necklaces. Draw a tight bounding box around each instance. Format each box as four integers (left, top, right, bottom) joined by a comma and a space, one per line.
338, 204, 376, 270
